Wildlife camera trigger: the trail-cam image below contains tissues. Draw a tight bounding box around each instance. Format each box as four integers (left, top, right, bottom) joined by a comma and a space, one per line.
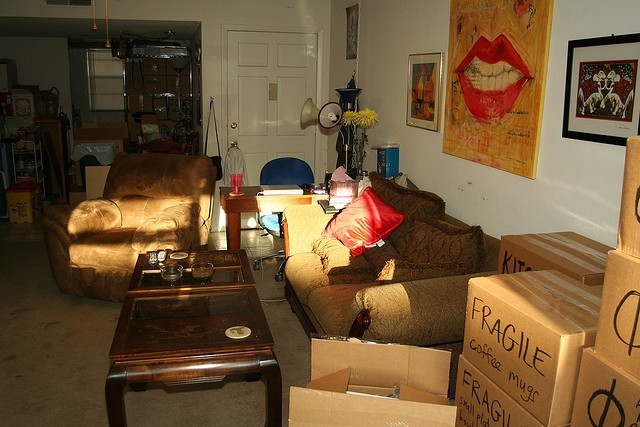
327, 167, 357, 196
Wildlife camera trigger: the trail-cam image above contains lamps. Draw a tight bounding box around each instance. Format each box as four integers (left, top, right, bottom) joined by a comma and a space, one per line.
318, 101, 354, 181
106, 0, 111, 48
90, 0, 98, 31
299, 97, 345, 175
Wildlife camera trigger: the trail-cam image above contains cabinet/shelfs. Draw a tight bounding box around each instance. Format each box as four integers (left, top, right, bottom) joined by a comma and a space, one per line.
30, 117, 67, 203
123, 55, 195, 152
11, 137, 46, 203
75, 126, 127, 153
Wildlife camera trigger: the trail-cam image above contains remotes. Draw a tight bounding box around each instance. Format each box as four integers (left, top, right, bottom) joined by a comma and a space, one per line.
148, 251, 157, 266
158, 250, 168, 262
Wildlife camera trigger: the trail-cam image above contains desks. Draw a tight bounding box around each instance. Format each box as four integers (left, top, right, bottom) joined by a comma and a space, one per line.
218, 183, 328, 251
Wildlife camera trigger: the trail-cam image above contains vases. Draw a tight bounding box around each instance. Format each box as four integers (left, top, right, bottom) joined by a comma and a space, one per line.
346, 170, 368, 181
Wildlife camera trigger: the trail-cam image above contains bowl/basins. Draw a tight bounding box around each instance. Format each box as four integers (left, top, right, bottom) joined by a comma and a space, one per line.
191, 262, 214, 281
160, 263, 184, 282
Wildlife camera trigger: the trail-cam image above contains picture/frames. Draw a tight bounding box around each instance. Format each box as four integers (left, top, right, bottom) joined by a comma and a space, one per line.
343, 2, 358, 62
561, 32, 639, 147
441, 0, 554, 180
405, 51, 444, 133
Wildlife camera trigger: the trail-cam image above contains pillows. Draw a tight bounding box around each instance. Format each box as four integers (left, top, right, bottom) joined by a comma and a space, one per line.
311, 237, 371, 276
320, 185, 406, 257
373, 257, 469, 285
406, 211, 485, 274
369, 172, 446, 248
362, 237, 402, 274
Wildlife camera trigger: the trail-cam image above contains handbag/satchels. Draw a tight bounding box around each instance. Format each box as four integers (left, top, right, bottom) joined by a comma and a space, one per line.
205, 101, 222, 181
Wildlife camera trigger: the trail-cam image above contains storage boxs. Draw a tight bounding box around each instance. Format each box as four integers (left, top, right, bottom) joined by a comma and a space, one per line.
4, 181, 40, 224
592, 250, 636, 379
462, 268, 601, 427
570, 345, 636, 426
452, 355, 547, 427
498, 231, 619, 286
288, 336, 458, 427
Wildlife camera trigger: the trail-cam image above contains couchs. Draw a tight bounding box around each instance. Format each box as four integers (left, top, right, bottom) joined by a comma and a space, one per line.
38, 151, 217, 304
280, 177, 501, 400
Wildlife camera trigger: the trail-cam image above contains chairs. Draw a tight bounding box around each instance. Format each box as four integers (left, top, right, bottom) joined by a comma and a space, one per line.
252, 156, 315, 283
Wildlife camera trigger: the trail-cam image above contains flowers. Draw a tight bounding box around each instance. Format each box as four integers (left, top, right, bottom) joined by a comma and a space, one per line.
342, 109, 380, 175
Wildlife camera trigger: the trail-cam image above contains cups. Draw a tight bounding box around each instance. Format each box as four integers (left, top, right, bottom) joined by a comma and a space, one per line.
229, 173, 243, 194
